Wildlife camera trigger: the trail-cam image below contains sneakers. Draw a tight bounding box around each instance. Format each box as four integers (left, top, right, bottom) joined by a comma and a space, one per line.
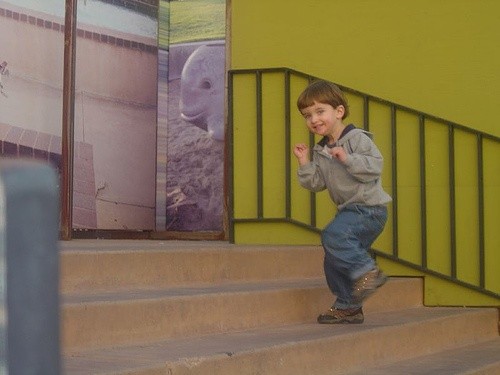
317, 305, 364, 324
351, 266, 387, 305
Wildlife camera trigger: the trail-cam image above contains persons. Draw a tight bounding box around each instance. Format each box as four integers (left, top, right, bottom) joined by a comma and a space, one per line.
291, 79, 393, 326
